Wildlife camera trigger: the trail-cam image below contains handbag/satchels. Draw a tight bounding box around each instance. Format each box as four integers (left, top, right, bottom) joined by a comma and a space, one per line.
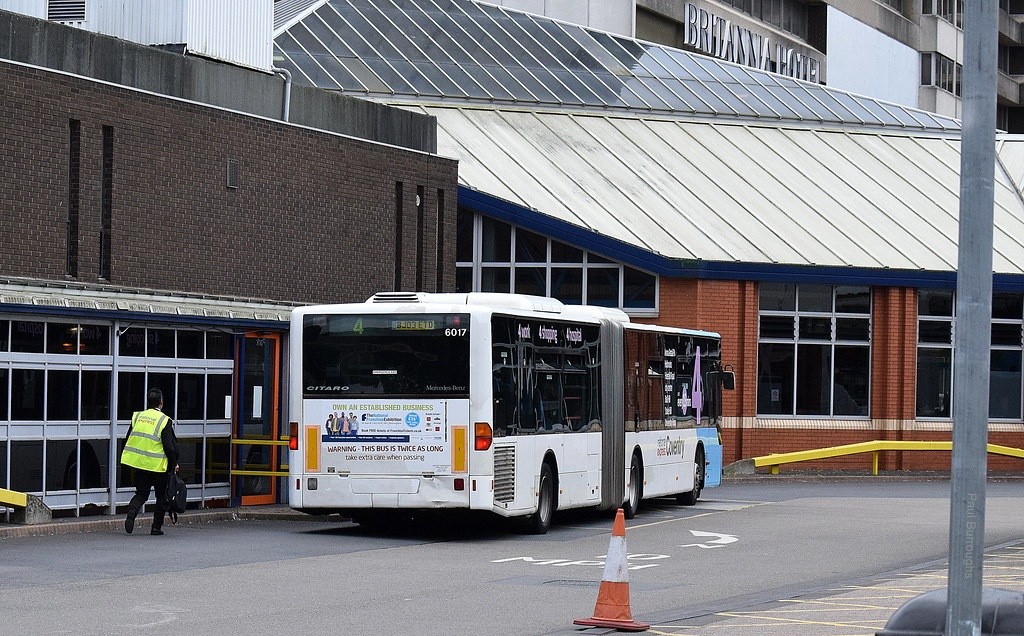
160, 468, 187, 526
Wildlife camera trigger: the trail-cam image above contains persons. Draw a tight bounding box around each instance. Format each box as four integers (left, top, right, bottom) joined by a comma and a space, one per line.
121, 387, 179, 535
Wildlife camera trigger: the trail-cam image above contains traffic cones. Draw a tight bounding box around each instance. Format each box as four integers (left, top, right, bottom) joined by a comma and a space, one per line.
572, 509, 651, 633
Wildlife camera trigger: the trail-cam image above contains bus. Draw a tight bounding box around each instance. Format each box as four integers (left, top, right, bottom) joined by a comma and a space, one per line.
282, 290, 737, 535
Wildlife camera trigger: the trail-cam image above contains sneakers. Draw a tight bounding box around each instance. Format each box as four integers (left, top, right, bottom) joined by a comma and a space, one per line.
125, 509, 136, 534
151, 527, 164, 535
